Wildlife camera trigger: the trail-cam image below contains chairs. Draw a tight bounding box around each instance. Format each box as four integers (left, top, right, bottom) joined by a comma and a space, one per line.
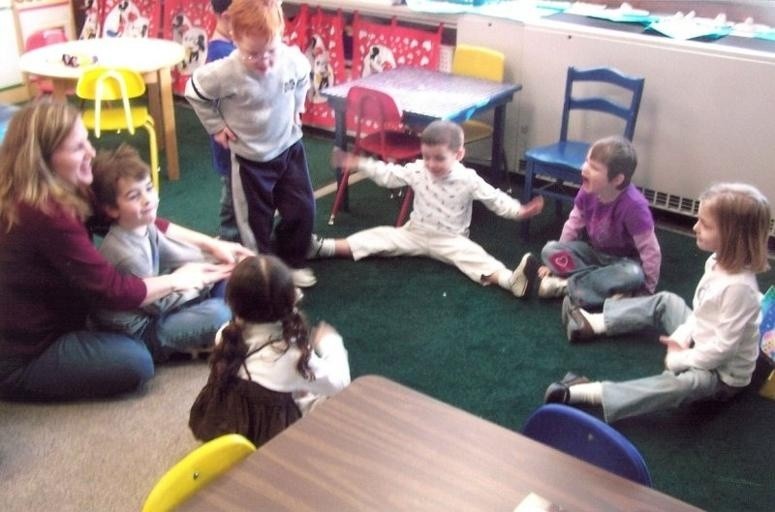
23, 27, 77, 104
519, 403, 651, 490
451, 44, 506, 193
522, 65, 645, 244
327, 86, 422, 226
75, 65, 159, 203
142, 433, 258, 512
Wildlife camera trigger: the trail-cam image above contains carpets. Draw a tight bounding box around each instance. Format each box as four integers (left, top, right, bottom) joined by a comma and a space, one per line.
13, 94, 355, 239
236, 162, 775, 512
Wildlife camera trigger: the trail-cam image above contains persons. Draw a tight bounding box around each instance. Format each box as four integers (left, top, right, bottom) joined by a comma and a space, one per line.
184, 0, 317, 288
204, 0, 242, 242
0, 97, 256, 403
543, 183, 772, 424
187, 254, 352, 450
537, 137, 662, 311
87, 147, 239, 356
306, 121, 545, 300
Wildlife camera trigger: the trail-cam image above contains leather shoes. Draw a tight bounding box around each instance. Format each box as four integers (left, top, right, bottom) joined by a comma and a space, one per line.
561, 295, 596, 345
543, 369, 589, 404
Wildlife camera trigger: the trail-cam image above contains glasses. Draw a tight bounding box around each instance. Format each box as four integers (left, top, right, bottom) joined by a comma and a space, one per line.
234, 30, 284, 65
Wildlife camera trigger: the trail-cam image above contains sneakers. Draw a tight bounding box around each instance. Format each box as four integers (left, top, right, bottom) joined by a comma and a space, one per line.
304, 233, 323, 258
509, 250, 537, 301
539, 275, 572, 299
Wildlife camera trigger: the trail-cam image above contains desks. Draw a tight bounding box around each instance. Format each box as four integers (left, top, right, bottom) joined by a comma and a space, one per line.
18, 36, 187, 181
319, 65, 522, 211
168, 373, 707, 512
